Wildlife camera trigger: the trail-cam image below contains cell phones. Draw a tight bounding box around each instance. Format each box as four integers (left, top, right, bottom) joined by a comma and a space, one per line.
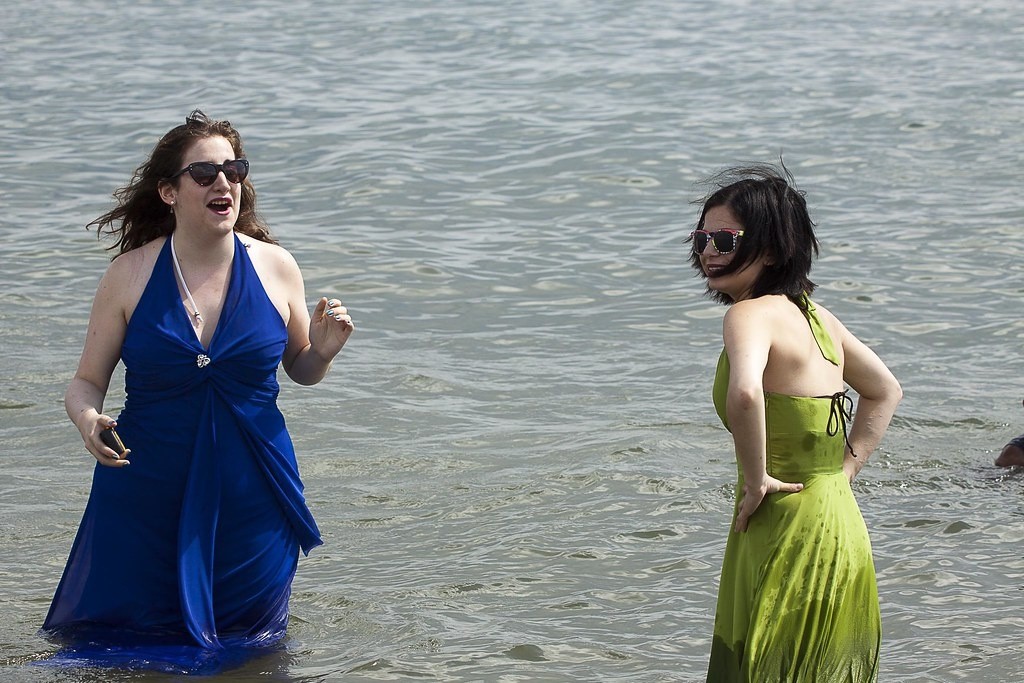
100, 428, 125, 455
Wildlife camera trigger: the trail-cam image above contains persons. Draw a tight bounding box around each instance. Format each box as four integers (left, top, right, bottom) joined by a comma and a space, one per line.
681, 173, 901, 681
60, 117, 356, 663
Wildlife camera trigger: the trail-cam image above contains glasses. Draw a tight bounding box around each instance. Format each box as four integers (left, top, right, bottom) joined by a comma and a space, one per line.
169, 159, 249, 188
689, 230, 744, 255
